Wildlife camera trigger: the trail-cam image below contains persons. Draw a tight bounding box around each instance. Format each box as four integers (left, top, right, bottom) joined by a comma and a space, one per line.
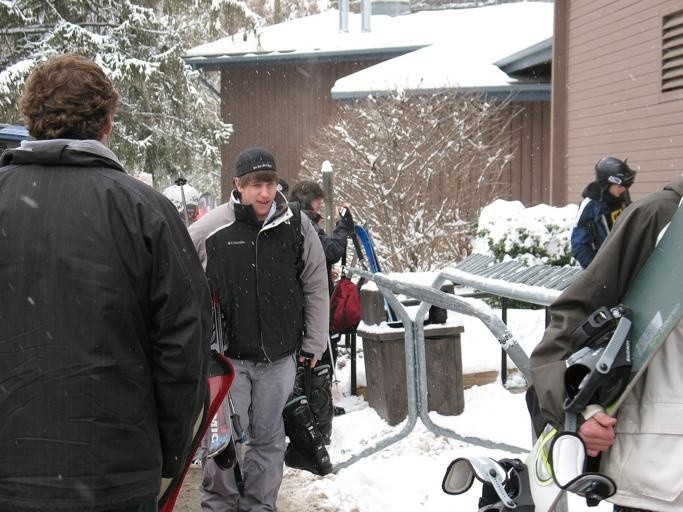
527, 180, 683, 510
568, 154, 635, 273
0, 54, 223, 510
185, 145, 329, 512
291, 178, 354, 476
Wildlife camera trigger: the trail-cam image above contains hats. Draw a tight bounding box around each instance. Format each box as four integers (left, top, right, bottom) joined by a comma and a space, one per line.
235, 147, 277, 178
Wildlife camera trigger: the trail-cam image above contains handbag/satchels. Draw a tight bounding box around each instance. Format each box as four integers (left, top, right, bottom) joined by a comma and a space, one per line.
329, 276, 361, 333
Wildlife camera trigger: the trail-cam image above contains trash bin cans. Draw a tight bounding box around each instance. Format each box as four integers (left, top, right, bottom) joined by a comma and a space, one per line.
357, 285, 464, 426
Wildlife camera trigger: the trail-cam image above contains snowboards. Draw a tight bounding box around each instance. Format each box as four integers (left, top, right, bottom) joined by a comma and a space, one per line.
534, 194, 683, 485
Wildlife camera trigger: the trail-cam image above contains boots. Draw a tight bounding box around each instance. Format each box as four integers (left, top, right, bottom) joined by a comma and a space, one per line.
282, 366, 334, 476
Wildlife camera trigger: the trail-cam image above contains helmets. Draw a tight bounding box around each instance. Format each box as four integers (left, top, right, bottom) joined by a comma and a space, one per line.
595, 155, 637, 191
